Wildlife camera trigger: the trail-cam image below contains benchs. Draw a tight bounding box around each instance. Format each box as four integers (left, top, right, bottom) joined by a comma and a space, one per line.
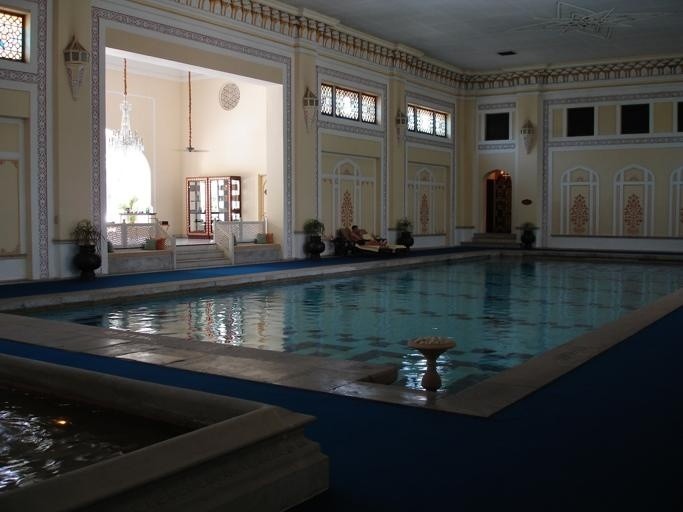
233, 242, 282, 265
107, 246, 171, 275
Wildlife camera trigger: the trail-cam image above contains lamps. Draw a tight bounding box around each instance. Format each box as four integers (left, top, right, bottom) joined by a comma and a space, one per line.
393, 106, 408, 147
59, 28, 91, 102
518, 119, 536, 156
104, 57, 146, 154
300, 86, 320, 136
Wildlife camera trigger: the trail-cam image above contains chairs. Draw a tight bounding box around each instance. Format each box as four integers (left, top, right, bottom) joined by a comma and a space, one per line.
358, 228, 407, 257
338, 226, 393, 260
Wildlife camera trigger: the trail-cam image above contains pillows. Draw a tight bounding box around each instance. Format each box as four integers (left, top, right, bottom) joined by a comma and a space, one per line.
142, 238, 157, 250
254, 232, 268, 244
264, 233, 274, 243
106, 240, 114, 253
149, 236, 166, 250
232, 234, 237, 247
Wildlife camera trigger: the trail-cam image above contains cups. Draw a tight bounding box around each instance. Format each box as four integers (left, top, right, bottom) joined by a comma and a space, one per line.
142, 237, 165, 250
256, 233, 273, 243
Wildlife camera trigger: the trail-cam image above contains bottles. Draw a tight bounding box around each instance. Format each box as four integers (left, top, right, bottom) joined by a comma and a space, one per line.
131, 207, 149, 213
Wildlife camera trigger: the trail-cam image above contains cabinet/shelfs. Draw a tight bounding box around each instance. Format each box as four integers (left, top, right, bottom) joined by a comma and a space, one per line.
182, 175, 243, 242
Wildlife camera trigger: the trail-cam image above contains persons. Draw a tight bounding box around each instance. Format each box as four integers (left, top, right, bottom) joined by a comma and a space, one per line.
350, 225, 387, 247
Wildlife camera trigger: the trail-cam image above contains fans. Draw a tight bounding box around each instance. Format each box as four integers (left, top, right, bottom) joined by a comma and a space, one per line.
170, 69, 211, 157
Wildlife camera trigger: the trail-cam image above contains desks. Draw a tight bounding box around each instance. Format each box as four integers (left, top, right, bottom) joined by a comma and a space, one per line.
118, 209, 157, 224
328, 237, 357, 259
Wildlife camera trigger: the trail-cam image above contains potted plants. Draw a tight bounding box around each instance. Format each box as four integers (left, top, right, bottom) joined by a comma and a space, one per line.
393, 214, 415, 252
66, 214, 104, 283
300, 216, 331, 262
